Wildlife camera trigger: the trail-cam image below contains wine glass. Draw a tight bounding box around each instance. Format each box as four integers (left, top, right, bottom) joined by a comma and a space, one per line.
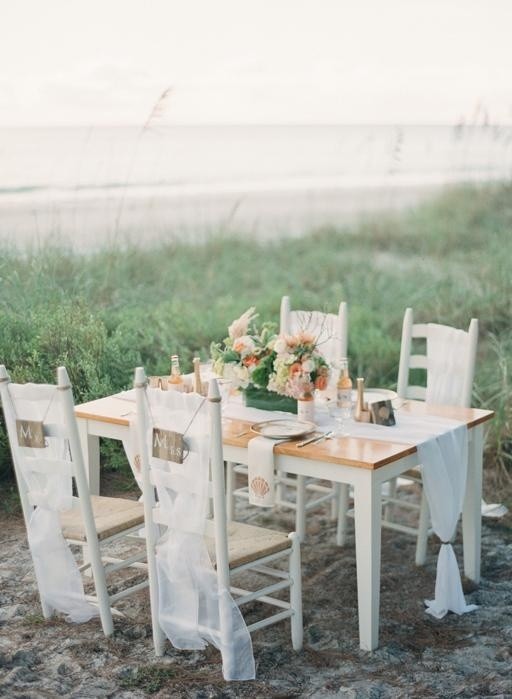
328, 403, 351, 438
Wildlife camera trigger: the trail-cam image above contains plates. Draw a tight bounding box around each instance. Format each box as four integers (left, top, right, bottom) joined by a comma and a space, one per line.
250, 419, 317, 438
352, 388, 397, 402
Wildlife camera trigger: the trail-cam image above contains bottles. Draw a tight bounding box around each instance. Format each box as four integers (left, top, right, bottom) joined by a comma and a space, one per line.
298, 371, 315, 419
167, 356, 184, 392
335, 358, 351, 403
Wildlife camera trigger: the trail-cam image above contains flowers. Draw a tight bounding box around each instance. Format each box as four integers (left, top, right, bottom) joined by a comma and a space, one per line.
209, 306, 330, 401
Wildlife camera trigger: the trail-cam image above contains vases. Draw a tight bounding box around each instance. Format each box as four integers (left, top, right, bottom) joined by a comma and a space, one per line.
243, 387, 297, 414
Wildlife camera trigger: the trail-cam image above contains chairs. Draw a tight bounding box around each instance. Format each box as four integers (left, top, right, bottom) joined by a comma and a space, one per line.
134, 367, 301, 682
0, 364, 145, 635
336, 309, 481, 568
227, 294, 347, 547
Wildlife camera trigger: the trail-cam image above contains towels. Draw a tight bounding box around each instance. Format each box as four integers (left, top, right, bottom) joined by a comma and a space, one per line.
248, 437, 294, 507
